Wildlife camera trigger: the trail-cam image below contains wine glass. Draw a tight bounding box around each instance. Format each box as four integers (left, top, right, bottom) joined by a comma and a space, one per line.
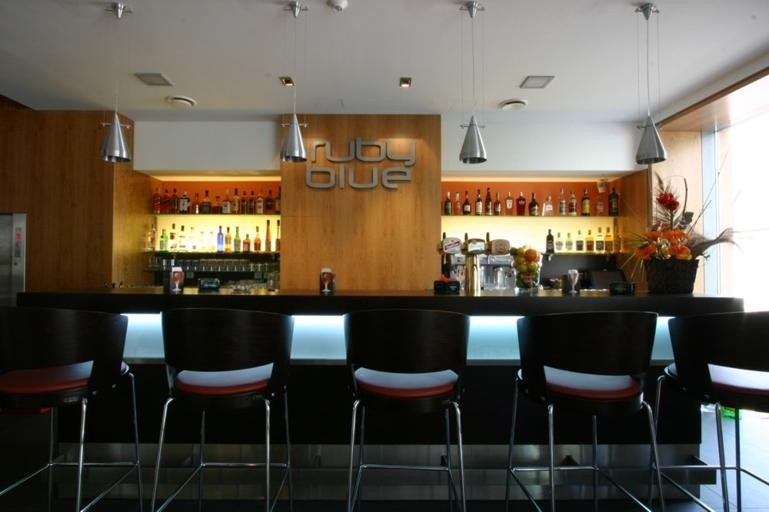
567, 268, 579, 295
320, 267, 333, 294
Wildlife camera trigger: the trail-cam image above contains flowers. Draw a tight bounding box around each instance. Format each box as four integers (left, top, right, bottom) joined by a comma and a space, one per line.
623, 152, 748, 279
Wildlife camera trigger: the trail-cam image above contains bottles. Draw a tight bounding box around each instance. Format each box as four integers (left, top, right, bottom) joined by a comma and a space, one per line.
141, 183, 282, 253
440, 187, 621, 254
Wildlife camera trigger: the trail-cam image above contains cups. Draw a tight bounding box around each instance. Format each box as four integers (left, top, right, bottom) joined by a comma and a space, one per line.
142, 255, 278, 294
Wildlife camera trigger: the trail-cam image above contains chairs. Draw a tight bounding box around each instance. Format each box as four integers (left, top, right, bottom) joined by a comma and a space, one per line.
0, 299, 145, 511
344, 305, 478, 510
648, 310, 768, 512
150, 307, 293, 512
504, 305, 665, 512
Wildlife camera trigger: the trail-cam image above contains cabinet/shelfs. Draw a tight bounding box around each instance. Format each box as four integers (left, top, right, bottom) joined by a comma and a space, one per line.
440, 173, 626, 261
141, 170, 282, 288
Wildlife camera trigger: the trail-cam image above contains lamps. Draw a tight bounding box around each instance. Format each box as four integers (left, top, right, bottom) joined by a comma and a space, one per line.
457, 1, 489, 167
279, 1, 308, 163
100, 3, 131, 165
633, 0, 668, 165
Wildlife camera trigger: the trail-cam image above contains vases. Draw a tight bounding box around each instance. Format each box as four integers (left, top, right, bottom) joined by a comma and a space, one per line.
642, 258, 699, 295
